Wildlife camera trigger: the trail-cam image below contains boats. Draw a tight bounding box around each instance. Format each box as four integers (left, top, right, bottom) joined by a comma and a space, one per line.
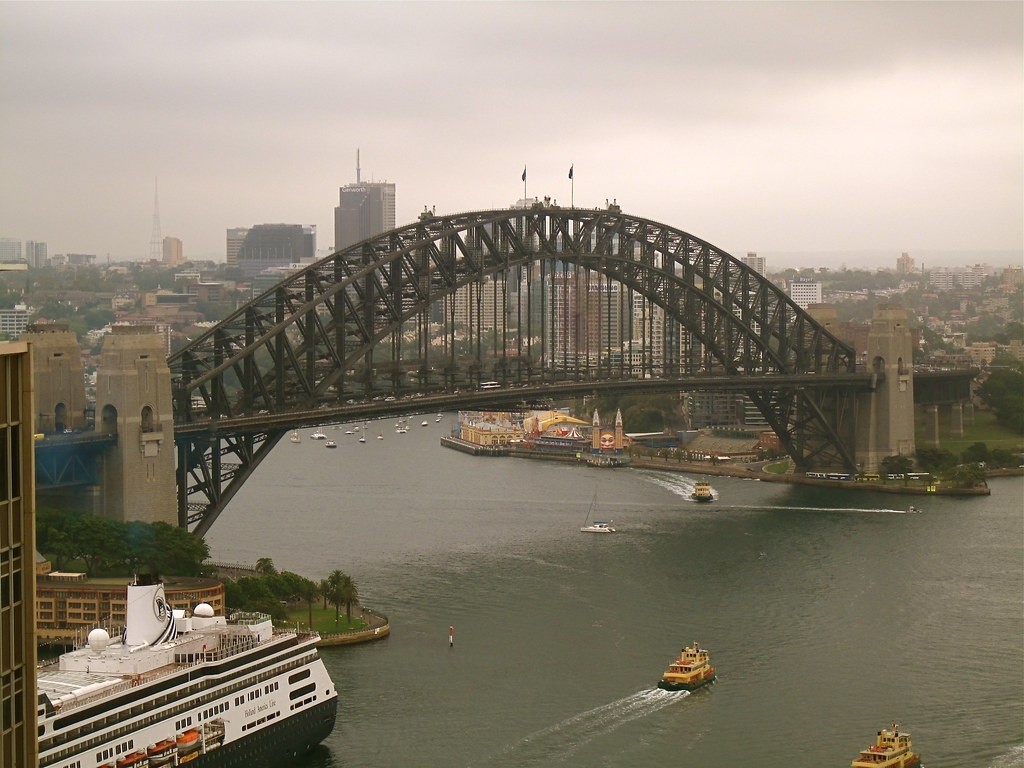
420, 419, 428, 426
394, 416, 414, 433
580, 482, 616, 533
659, 642, 717, 691
691, 479, 713, 501
436, 413, 444, 423
376, 434, 384, 440
291, 429, 300, 443
325, 439, 337, 448
37, 570, 338, 768
310, 424, 327, 440
850, 722, 923, 768
333, 419, 368, 442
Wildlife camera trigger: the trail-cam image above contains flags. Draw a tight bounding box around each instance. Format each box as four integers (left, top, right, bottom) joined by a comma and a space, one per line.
569, 167, 572, 179
522, 169, 526, 181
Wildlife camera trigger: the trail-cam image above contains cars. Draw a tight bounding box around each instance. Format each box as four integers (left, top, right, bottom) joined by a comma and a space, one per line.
346, 391, 425, 405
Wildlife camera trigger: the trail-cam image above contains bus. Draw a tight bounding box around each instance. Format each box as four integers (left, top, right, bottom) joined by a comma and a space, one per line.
477, 381, 501, 391
